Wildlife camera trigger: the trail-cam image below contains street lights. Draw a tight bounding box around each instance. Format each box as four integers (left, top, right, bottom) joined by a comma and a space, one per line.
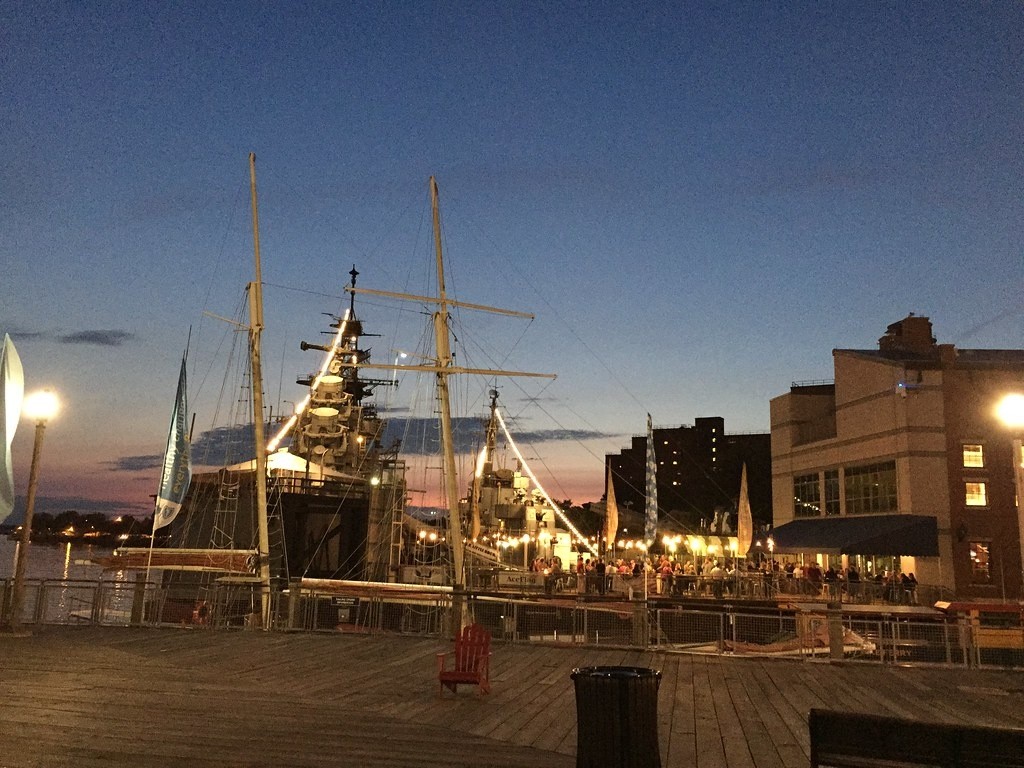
1, 391, 60, 634
993, 391, 1024, 571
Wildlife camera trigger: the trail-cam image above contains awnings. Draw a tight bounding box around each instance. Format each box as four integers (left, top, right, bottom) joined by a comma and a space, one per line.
750, 515, 940, 558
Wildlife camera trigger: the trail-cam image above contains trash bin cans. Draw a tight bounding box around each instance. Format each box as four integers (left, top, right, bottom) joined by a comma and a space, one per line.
568, 665, 664, 768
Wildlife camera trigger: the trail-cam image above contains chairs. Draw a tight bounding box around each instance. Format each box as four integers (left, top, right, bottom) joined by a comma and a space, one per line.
436, 623, 491, 699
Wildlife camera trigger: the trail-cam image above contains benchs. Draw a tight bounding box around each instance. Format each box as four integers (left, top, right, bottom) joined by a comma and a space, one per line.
807, 708, 1024, 768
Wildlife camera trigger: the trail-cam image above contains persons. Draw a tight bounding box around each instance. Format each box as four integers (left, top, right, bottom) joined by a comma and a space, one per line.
528, 551, 918, 606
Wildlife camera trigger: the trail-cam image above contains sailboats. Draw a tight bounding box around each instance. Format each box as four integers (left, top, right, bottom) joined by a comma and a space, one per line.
68, 152, 878, 657
